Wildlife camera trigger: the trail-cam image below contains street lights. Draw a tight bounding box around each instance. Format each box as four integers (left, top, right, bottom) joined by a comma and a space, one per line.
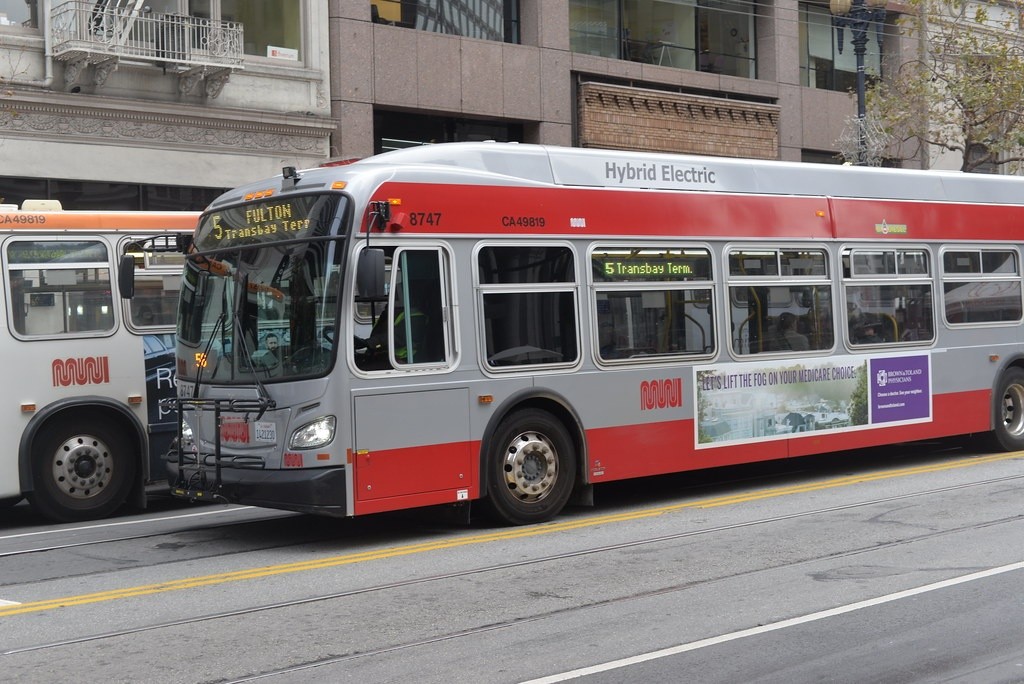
828, 0, 888, 167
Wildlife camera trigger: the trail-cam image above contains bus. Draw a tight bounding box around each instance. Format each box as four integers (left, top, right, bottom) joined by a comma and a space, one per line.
0, 199, 398, 522
116, 137, 1024, 529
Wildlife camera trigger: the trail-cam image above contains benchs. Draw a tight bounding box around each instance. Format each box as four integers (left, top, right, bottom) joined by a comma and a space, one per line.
646, 40, 674, 65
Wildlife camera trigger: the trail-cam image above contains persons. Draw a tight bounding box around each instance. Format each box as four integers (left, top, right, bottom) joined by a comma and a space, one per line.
361, 283, 424, 363
265, 334, 278, 350
770, 312, 809, 352
